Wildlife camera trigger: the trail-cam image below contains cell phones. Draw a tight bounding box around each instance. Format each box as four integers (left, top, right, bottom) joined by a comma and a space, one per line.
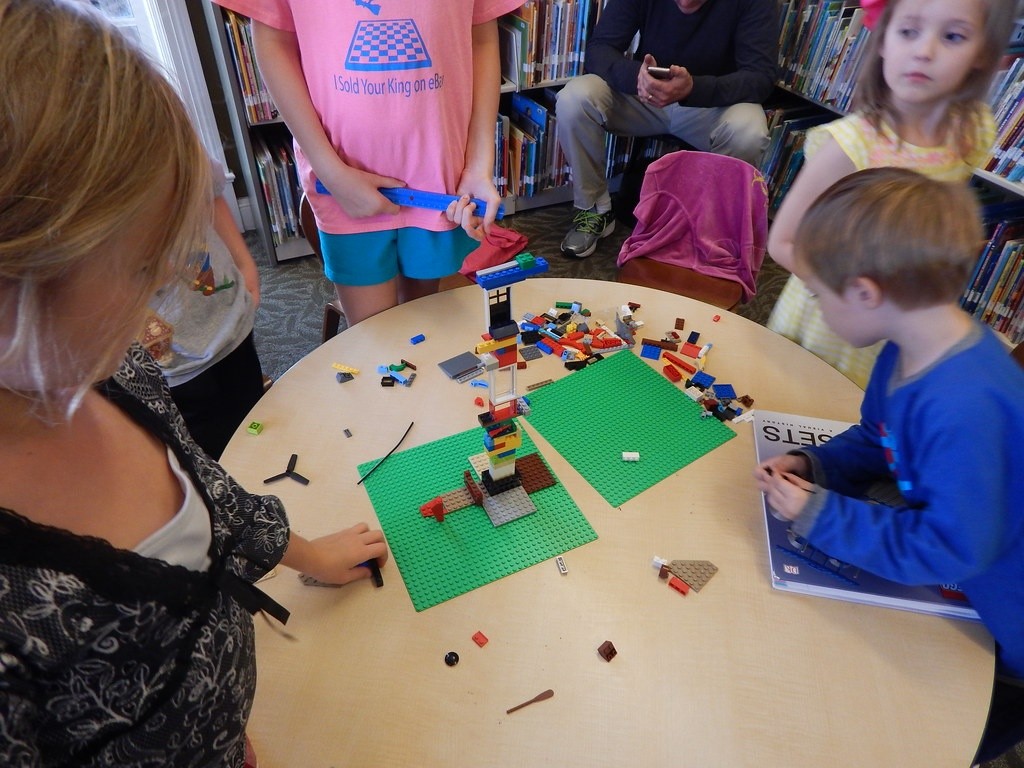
647, 66, 673, 80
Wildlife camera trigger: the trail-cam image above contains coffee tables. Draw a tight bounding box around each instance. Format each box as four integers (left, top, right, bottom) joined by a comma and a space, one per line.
210, 277, 997, 768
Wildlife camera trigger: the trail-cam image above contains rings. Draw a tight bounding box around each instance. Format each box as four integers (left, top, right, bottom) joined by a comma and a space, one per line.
647, 94, 654, 101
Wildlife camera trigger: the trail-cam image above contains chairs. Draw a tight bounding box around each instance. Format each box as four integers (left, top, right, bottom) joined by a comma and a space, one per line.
615, 150, 770, 315
299, 191, 348, 343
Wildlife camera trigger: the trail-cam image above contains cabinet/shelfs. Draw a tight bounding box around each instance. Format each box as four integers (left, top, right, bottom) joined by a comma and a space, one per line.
205, 0, 1024, 362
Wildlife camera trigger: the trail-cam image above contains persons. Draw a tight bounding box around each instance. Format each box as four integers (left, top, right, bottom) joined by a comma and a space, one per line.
1, 2, 389, 768
556, 0, 781, 259
753, 169, 1024, 768
211, 1, 529, 328
763, 2, 1017, 392
131, 163, 269, 462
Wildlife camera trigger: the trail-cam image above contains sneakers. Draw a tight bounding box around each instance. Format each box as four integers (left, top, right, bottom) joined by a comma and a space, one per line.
560, 201, 615, 257
